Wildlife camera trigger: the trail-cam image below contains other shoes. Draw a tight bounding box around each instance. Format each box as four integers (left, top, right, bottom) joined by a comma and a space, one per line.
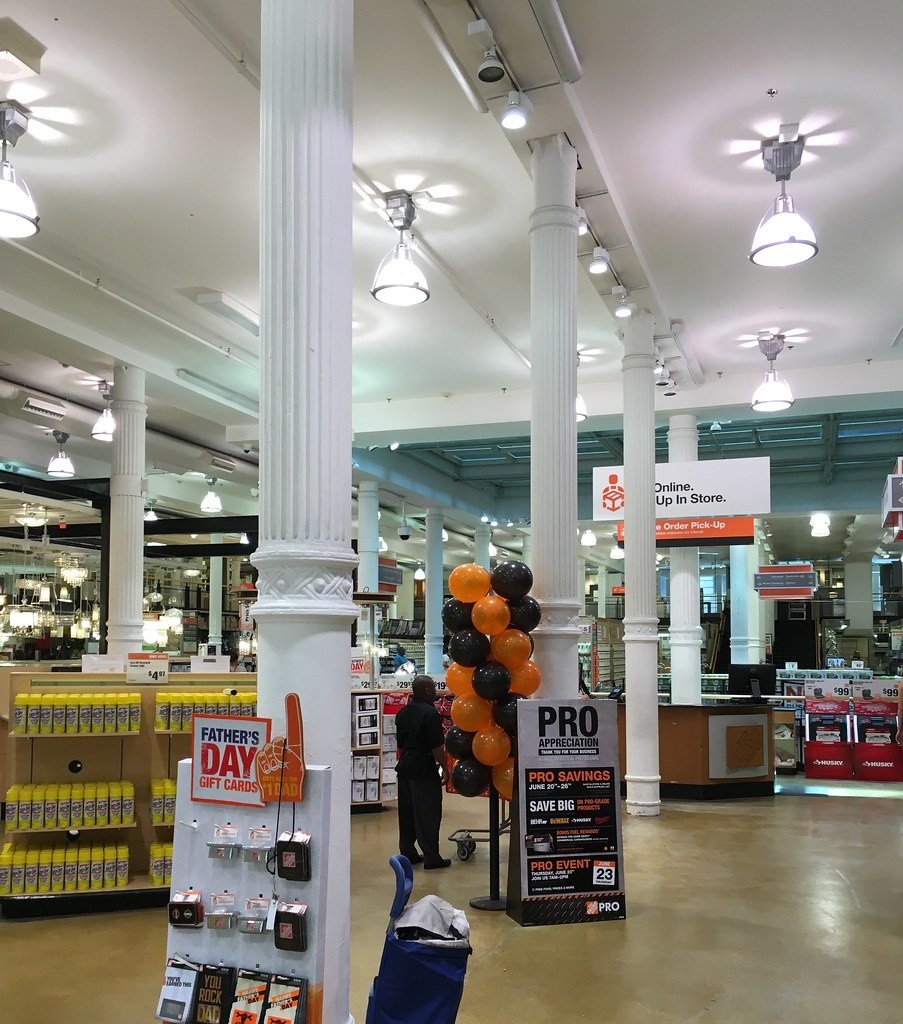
424, 858, 452, 870
410, 854, 424, 864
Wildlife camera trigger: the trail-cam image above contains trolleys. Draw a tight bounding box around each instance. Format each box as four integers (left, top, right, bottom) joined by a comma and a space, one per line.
448, 785, 511, 861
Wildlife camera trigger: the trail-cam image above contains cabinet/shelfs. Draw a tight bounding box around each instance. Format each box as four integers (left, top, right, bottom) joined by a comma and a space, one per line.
0, 616, 802, 925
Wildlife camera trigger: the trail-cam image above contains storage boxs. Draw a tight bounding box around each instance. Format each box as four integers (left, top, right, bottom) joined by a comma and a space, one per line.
381, 784, 398, 801
365, 754, 380, 780
383, 715, 396, 734
351, 695, 379, 714
366, 779, 378, 801
383, 733, 397, 752
351, 781, 364, 802
351, 712, 380, 731
382, 752, 397, 768
353, 756, 367, 782
351, 729, 381, 748
382, 769, 397, 784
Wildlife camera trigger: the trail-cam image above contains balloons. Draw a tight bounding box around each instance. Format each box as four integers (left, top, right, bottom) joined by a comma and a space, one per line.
441, 561, 542, 799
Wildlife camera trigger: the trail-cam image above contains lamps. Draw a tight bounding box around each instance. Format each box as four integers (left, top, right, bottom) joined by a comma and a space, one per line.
764, 530, 773, 537
655, 368, 670, 386
412, 512, 663, 580
750, 332, 795, 412
369, 188, 430, 307
500, 91, 527, 130
808, 513, 831, 538
588, 247, 609, 275
611, 285, 632, 318
664, 380, 676, 397
576, 351, 587, 423
710, 421, 721, 431
746, 134, 819, 267
881, 551, 890, 560
468, 20, 506, 83
578, 218, 588, 235
0, 382, 387, 649
0, 103, 41, 239
763, 521, 771, 528
654, 363, 663, 374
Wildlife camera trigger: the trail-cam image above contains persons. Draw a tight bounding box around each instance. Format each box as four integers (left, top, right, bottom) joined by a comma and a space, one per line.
395, 676, 452, 869
393, 647, 407, 673
443, 649, 450, 669
846, 651, 864, 667
578, 663, 595, 699
229, 649, 247, 672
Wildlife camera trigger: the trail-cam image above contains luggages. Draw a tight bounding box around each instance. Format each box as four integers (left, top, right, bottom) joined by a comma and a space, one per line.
364, 857, 473, 1024
